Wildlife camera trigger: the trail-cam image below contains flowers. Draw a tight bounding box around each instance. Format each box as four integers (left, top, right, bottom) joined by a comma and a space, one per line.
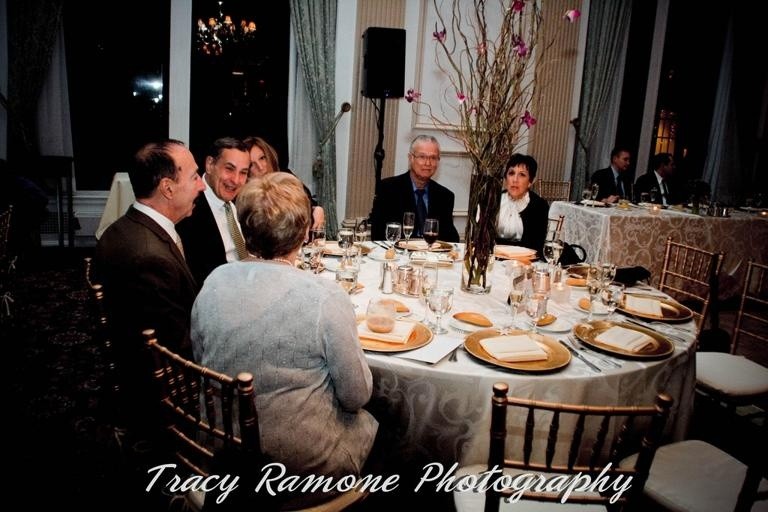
401, 1, 588, 278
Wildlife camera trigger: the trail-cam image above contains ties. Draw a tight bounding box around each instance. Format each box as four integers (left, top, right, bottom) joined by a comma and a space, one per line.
616, 175, 624, 199
224, 201, 249, 260
661, 180, 667, 200
416, 190, 428, 237
176, 232, 186, 261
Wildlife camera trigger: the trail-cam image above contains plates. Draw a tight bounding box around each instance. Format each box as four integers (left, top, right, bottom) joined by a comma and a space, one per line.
575, 319, 675, 360
462, 329, 571, 375
611, 288, 695, 327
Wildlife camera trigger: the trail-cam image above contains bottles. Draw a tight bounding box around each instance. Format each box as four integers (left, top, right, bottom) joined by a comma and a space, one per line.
573, 181, 766, 216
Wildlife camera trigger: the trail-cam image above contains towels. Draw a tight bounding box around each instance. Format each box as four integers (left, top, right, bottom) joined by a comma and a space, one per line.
323, 237, 535, 261
595, 324, 652, 353
476, 334, 548, 364
358, 318, 414, 344
623, 294, 664, 318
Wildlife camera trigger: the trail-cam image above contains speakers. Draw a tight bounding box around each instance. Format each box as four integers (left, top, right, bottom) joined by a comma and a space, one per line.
360, 27, 405, 99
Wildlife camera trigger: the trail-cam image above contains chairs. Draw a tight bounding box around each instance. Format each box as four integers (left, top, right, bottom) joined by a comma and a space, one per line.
459, 372, 672, 510
543, 215, 564, 244
85, 258, 162, 460
652, 234, 735, 347
683, 261, 768, 417
136, 325, 380, 510
537, 175, 579, 204
611, 413, 768, 512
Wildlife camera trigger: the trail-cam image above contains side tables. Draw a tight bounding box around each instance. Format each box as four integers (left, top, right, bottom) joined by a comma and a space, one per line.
266, 234, 707, 470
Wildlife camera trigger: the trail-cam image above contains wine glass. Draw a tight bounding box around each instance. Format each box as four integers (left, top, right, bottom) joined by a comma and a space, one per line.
297, 209, 626, 354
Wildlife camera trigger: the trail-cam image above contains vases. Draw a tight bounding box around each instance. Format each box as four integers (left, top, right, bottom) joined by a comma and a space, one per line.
461, 155, 511, 297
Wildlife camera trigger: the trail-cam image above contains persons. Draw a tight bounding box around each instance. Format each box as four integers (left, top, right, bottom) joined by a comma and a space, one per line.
82, 140, 203, 432
634, 152, 676, 206
585, 146, 635, 206
185, 169, 411, 511
176, 136, 251, 294
492, 153, 550, 250
365, 133, 460, 242
239, 130, 314, 244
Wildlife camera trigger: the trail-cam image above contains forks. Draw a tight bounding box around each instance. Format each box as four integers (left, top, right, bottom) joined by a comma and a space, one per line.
565, 332, 621, 369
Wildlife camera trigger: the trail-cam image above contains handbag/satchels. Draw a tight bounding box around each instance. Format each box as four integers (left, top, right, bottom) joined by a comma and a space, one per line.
541, 240, 586, 265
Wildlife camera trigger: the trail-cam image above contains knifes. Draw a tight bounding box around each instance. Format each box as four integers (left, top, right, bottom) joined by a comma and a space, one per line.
557, 336, 603, 377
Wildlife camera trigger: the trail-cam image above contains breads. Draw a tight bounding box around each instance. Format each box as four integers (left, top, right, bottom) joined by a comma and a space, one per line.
579, 298, 591, 310
566, 278, 586, 287
381, 299, 409, 312
536, 314, 557, 326
453, 312, 493, 327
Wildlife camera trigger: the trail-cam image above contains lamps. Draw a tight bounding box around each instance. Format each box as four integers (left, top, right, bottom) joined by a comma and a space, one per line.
191, 2, 260, 59
